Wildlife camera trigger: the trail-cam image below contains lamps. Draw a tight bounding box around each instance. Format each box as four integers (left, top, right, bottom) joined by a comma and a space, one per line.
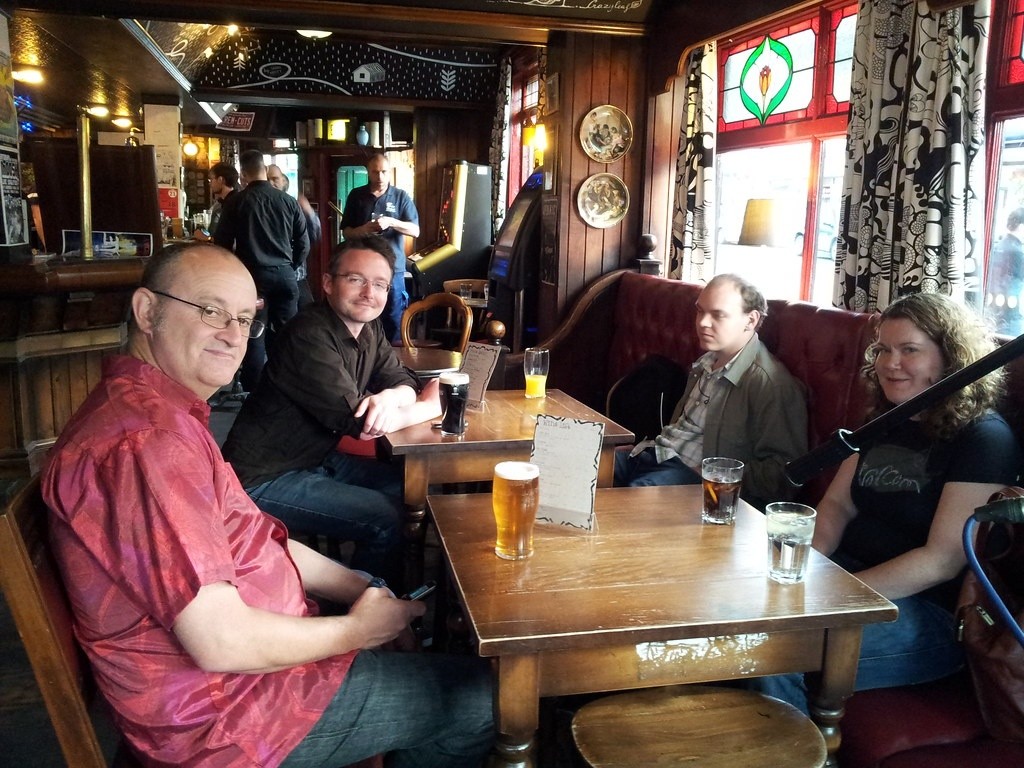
297, 30, 332, 41
125, 127, 144, 147
522, 105, 548, 152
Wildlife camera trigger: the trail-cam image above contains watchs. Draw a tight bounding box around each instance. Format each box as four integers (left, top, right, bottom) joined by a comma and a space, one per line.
207, 236, 212, 240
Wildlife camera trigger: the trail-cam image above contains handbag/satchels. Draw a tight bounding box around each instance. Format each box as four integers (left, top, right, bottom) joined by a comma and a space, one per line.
605, 355, 686, 446
954, 484, 1024, 742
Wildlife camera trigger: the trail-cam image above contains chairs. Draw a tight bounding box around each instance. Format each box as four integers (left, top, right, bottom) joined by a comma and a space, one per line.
401, 279, 489, 356
0, 463, 386, 767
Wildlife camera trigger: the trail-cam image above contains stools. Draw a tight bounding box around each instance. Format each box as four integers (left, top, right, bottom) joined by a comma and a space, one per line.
572, 686, 827, 768
208, 295, 265, 407
392, 339, 442, 348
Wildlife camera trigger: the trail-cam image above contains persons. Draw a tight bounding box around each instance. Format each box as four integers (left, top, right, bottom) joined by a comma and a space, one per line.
984, 206, 1024, 337
220, 231, 444, 597
340, 153, 421, 346
737, 291, 1018, 717
194, 151, 322, 392
613, 274, 810, 488
40, 240, 501, 768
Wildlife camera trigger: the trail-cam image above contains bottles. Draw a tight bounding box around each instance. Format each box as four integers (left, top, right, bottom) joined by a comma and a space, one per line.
160, 211, 165, 238
167, 225, 173, 238
356, 125, 369, 145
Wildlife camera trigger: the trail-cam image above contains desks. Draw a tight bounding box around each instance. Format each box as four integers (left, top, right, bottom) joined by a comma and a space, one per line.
393, 347, 463, 389
427, 484, 899, 767
377, 390, 635, 593
464, 298, 488, 308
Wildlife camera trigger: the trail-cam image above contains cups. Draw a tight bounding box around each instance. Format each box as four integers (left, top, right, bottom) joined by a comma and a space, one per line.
440, 372, 469, 437
524, 348, 549, 400
193, 210, 212, 231
492, 462, 540, 563
372, 213, 383, 235
702, 457, 744, 524
461, 283, 472, 302
766, 501, 817, 585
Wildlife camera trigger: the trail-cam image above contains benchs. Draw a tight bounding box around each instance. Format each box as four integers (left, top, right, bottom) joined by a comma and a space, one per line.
489, 234, 1024, 768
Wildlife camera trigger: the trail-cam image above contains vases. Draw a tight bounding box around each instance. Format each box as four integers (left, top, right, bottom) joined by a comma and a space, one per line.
356, 125, 369, 145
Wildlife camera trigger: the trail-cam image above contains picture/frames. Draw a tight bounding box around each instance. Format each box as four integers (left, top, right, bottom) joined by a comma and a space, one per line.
545, 72, 559, 116
302, 179, 314, 199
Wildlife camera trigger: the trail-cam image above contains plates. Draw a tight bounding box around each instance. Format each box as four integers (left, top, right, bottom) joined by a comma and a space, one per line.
577, 172, 630, 228
579, 105, 633, 164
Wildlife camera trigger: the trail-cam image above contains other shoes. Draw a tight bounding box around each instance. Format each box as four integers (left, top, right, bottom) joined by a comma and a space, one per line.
414, 629, 434, 647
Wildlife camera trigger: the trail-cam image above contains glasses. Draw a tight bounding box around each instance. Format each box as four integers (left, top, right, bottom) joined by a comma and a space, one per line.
335, 273, 392, 295
151, 290, 264, 339
208, 177, 217, 182
